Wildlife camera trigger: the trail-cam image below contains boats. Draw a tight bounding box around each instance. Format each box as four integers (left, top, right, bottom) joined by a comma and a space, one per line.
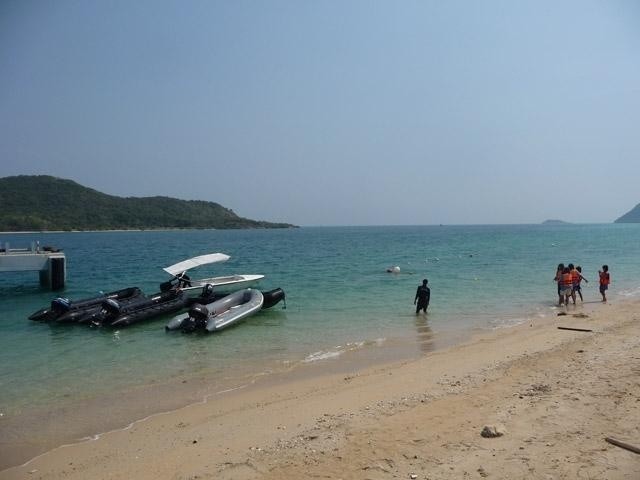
28, 253, 287, 335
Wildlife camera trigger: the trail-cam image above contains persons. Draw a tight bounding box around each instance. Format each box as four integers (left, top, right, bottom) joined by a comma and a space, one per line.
412, 279, 432, 314
177, 275, 192, 288
554, 263, 589, 311
598, 264, 612, 299
